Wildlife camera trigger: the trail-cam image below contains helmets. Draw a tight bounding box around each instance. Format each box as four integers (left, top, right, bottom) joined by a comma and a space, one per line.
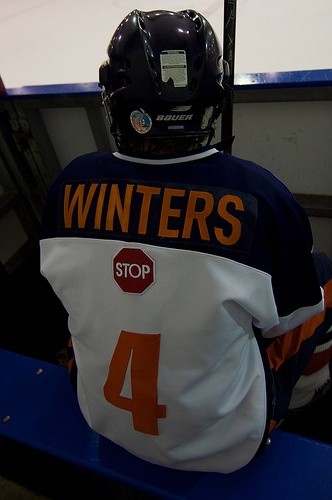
97, 9, 231, 155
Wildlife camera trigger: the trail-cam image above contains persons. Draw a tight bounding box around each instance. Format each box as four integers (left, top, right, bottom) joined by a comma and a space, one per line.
38, 7, 332, 478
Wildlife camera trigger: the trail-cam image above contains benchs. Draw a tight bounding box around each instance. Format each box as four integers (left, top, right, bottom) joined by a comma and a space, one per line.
0, 349, 332, 500
